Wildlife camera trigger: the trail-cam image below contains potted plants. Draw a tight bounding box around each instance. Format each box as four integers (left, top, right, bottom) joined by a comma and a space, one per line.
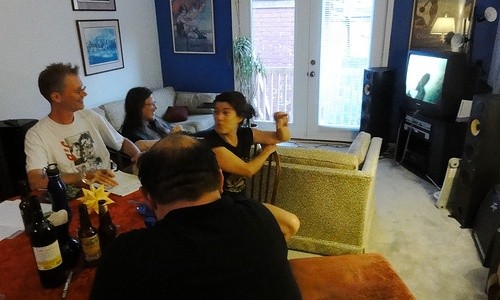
233, 35, 268, 130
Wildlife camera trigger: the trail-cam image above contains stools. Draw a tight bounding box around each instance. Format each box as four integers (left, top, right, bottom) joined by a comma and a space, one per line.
286, 252, 417, 300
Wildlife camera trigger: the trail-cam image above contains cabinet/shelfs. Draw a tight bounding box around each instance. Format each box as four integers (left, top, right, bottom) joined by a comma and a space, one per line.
393, 114, 450, 184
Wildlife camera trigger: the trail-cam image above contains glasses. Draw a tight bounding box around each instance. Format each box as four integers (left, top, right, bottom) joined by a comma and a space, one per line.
58, 86, 87, 93
143, 101, 157, 107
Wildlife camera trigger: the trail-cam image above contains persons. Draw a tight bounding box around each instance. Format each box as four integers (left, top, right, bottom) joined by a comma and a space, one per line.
24, 63, 142, 191
204, 90, 300, 237
121, 87, 187, 151
88, 131, 302, 300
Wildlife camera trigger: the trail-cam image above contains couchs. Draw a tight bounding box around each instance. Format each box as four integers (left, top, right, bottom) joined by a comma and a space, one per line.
90, 86, 222, 140
245, 130, 383, 255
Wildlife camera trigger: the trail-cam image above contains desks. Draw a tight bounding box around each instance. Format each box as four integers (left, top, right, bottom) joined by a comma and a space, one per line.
0, 185, 155, 300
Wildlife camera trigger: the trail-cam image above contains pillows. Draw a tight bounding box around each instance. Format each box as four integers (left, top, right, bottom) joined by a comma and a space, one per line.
163, 105, 189, 123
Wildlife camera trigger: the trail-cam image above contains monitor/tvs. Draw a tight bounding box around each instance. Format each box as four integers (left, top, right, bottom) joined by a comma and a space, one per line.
403, 47, 475, 118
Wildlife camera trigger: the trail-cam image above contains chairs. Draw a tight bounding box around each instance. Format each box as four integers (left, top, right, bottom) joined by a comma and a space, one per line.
0, 119, 137, 202
248, 142, 280, 205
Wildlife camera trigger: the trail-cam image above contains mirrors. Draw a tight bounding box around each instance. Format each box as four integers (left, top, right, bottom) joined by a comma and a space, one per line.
407, 0, 475, 51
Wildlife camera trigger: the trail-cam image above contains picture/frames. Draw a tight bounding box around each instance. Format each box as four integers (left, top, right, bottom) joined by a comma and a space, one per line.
170, 0, 215, 54
76, 18, 125, 76
71, 0, 117, 12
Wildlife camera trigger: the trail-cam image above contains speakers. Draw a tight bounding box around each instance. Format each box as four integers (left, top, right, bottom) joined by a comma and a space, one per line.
360, 66, 397, 156
472, 185, 500, 268
446, 94, 500, 228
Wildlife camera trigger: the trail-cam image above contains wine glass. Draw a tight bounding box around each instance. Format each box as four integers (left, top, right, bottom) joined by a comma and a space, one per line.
79, 163, 97, 191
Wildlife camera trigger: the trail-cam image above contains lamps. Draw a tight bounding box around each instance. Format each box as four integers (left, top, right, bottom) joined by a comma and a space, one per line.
429, 13, 455, 43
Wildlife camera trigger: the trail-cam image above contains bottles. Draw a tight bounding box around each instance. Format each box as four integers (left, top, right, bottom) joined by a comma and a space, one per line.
97, 200, 117, 249
19, 180, 33, 236
27, 195, 66, 288
46, 163, 69, 212
78, 203, 102, 267
